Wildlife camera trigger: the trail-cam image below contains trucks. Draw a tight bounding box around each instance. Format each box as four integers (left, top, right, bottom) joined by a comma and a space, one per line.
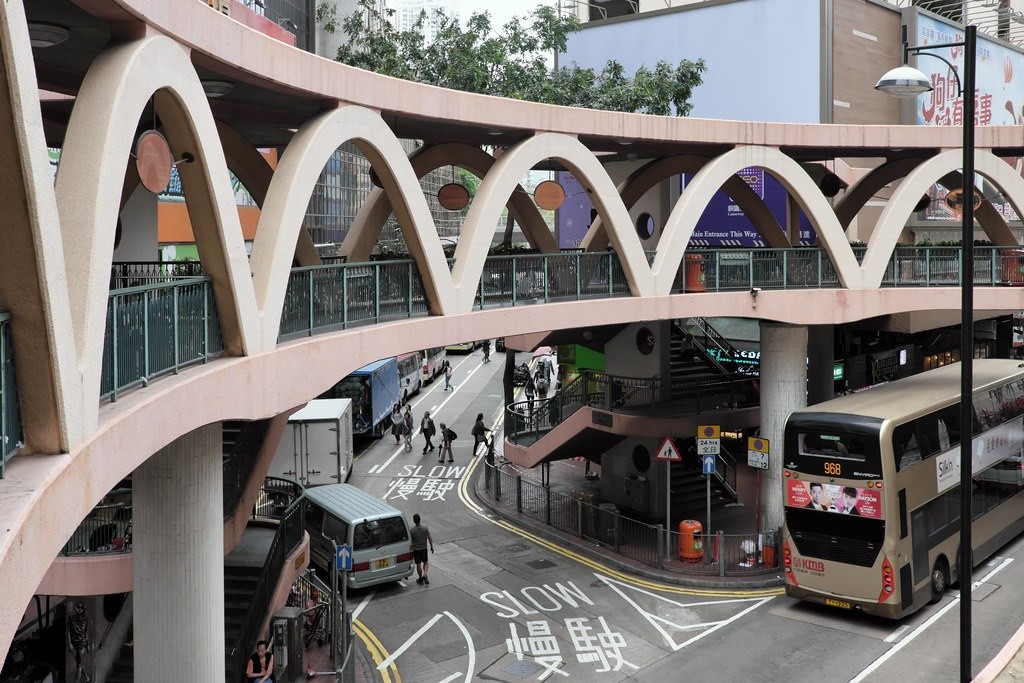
319, 354, 411, 438
262, 397, 355, 490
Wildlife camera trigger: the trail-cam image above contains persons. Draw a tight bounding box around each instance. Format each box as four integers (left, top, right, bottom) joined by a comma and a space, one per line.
246, 640, 276, 683
481, 339, 491, 364
839, 487, 861, 516
124, 525, 132, 550
409, 514, 434, 585
229, 470, 244, 496
471, 413, 492, 458
67, 600, 92, 683
441, 361, 454, 392
521, 363, 527, 367
525, 378, 537, 411
391, 403, 404, 445
402, 405, 414, 454
438, 423, 454, 463
537, 374, 548, 407
804, 482, 828, 512
419, 411, 436, 455
680, 333, 697, 362
871, 358, 882, 385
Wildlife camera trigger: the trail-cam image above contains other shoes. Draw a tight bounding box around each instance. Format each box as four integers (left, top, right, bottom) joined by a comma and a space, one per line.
487, 358, 491, 362
402, 451, 407, 454
423, 450, 426, 454
429, 447, 435, 451
473, 454, 477, 456
483, 358, 486, 363
444, 388, 447, 390
423, 574, 429, 584
452, 387, 454, 392
394, 443, 399, 445
408, 447, 413, 452
438, 460, 444, 463
447, 460, 454, 462
416, 579, 423, 585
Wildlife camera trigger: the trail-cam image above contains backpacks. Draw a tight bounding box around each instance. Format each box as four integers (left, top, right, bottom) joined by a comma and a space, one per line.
538, 380, 546, 395
447, 428, 457, 440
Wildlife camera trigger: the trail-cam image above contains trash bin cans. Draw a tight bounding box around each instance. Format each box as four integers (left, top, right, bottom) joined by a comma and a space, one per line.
599, 503, 621, 546
1002, 249, 1024, 286
678, 519, 704, 563
686, 254, 706, 292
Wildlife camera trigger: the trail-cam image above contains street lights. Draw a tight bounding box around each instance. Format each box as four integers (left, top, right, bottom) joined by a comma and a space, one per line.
871, 45, 976, 683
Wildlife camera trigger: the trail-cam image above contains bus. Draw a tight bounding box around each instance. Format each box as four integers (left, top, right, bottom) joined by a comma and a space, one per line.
395, 351, 424, 406
415, 345, 447, 385
778, 358, 1024, 621
444, 340, 486, 353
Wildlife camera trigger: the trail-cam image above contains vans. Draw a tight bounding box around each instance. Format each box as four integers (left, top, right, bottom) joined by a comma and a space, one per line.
298, 481, 416, 593
